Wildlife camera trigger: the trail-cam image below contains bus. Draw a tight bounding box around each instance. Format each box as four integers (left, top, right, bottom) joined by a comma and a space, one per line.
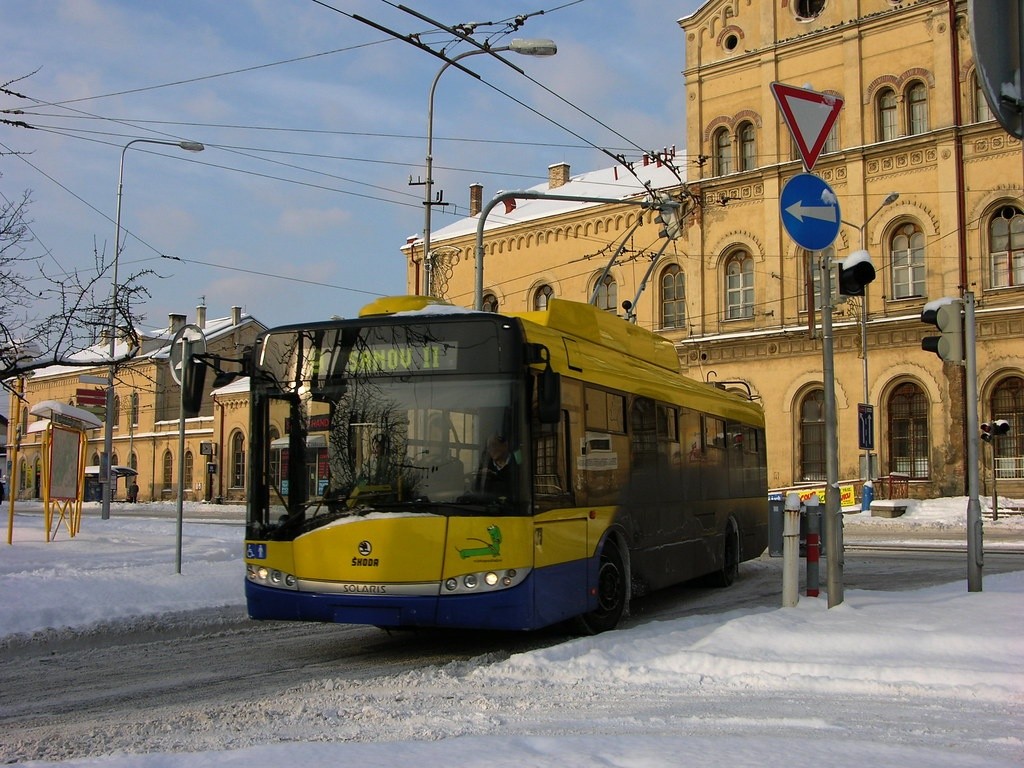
183, 291, 772, 637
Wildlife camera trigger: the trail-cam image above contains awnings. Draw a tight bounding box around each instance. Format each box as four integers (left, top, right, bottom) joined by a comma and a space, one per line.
271, 434, 326, 449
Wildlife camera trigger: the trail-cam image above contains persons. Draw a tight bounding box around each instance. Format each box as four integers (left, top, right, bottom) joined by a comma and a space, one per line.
0, 482, 4, 504
472, 433, 525, 499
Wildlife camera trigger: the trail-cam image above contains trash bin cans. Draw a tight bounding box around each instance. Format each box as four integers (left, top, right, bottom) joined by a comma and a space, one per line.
767, 500, 828, 557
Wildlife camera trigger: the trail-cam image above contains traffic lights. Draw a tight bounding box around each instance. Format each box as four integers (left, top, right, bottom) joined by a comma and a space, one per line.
980, 423, 992, 443
995, 420, 1010, 435
920, 301, 967, 367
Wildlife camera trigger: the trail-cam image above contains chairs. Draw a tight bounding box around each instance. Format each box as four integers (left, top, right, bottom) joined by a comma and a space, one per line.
415, 453, 464, 493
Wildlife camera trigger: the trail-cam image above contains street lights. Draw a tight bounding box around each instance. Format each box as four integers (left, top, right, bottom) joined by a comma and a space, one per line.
833, 188, 902, 477
420, 36, 560, 295
102, 139, 207, 520
